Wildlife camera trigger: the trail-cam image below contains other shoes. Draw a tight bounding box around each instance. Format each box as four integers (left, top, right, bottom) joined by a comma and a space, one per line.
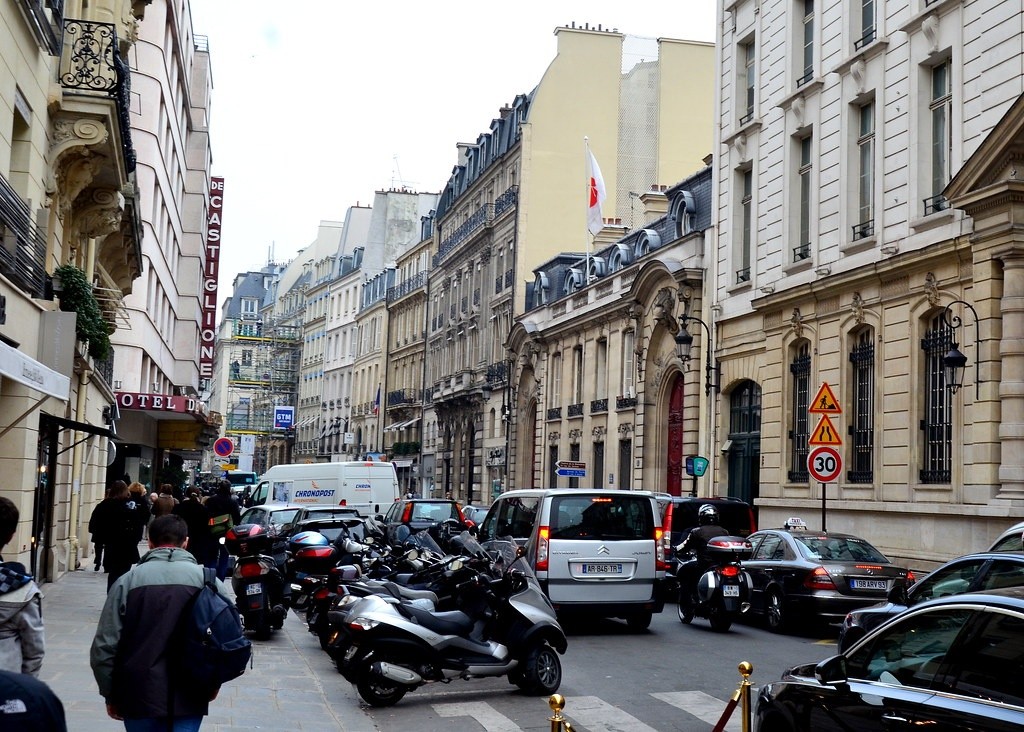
95, 563, 101, 571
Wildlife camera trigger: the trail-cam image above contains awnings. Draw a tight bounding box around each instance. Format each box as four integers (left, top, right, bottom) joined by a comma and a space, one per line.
38, 413, 124, 455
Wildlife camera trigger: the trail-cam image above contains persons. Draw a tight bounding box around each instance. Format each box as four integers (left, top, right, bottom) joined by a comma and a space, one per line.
402, 489, 417, 499
672, 504, 729, 603
0, 496, 46, 679
89, 514, 229, 732
88, 480, 242, 595
446, 492, 454, 500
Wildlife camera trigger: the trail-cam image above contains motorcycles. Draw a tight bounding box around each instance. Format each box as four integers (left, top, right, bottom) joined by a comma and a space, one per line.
287, 504, 568, 707
670, 535, 754, 633
219, 514, 292, 641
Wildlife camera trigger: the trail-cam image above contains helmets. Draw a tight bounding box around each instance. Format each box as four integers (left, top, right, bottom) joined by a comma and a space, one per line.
698, 504, 719, 526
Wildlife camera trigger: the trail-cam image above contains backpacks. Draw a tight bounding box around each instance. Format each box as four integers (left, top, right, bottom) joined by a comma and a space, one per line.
188, 567, 252, 685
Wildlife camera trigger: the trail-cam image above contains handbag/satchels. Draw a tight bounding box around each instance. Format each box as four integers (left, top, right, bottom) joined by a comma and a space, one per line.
208, 513, 234, 534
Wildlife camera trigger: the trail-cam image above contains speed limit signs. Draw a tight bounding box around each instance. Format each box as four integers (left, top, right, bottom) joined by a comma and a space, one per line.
807, 447, 843, 483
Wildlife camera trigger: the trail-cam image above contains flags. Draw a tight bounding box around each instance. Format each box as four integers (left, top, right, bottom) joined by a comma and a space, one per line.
588, 149, 607, 237
374, 388, 380, 415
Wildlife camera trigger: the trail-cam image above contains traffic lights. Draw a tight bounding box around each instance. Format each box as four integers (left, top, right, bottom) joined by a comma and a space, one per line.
197, 464, 201, 472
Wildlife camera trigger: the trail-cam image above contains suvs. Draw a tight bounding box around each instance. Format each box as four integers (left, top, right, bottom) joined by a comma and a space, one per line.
653, 491, 756, 603
374, 496, 466, 547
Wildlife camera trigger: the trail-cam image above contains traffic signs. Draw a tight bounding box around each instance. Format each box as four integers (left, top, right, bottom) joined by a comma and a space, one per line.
555, 469, 586, 477
555, 460, 586, 469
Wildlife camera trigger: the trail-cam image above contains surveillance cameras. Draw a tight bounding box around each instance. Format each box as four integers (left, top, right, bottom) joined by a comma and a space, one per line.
721, 439, 733, 453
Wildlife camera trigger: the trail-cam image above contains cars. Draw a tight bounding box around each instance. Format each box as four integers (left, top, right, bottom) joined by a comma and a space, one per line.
240, 504, 306, 525
461, 505, 491, 528
731, 518, 916, 633
931, 522, 1024, 598
291, 517, 372, 542
838, 552, 1024, 655
291, 505, 361, 529
754, 586, 1024, 732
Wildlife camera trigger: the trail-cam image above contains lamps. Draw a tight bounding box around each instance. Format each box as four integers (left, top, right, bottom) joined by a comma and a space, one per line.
939, 299, 980, 401
103, 402, 117, 425
673, 310, 721, 397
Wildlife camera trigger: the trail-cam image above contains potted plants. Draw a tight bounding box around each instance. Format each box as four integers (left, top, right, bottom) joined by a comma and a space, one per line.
52, 263, 112, 360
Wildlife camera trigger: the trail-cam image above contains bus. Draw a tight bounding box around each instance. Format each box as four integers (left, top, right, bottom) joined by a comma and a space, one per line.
200, 470, 258, 504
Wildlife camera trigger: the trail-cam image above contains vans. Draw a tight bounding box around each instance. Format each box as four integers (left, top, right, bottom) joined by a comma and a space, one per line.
469, 488, 665, 631
239, 461, 401, 529
